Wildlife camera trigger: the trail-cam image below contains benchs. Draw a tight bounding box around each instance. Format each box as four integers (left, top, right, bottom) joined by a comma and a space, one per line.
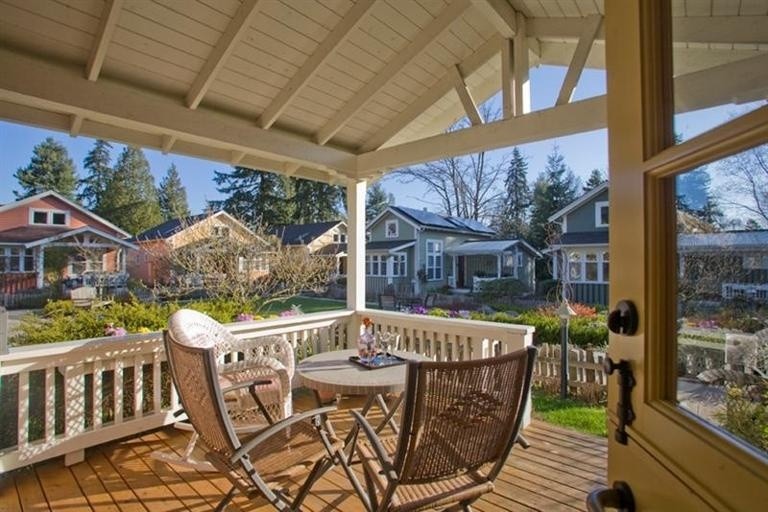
70, 286, 116, 310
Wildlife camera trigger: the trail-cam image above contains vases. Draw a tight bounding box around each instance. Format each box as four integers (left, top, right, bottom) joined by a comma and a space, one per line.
357, 329, 377, 362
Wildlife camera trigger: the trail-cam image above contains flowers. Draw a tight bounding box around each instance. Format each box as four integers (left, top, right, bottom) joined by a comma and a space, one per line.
362, 317, 374, 328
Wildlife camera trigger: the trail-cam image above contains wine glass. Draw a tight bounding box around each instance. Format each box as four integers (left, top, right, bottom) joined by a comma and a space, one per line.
378, 331, 400, 361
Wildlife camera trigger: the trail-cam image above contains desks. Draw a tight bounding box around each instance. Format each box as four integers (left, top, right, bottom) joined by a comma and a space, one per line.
296, 346, 434, 467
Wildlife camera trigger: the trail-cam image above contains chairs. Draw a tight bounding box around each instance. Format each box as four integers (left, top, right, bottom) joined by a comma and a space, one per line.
336, 345, 537, 512
378, 281, 438, 310
150, 308, 295, 472
161, 327, 346, 512
